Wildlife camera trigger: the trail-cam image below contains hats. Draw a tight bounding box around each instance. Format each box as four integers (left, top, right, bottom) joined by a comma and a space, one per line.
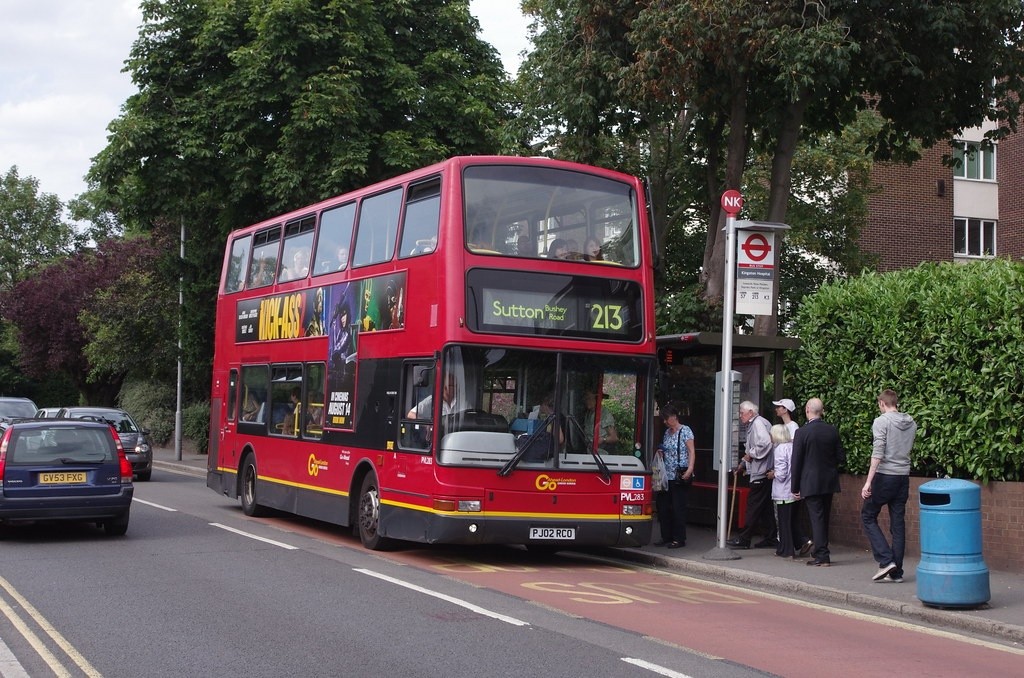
772, 399, 796, 412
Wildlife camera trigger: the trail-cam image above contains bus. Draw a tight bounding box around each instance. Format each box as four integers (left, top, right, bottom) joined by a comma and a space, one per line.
205, 155, 670, 556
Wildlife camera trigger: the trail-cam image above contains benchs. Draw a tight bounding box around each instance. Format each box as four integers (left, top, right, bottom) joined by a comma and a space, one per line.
688, 480, 751, 530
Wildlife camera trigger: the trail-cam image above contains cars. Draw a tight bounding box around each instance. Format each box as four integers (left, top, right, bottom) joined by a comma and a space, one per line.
34, 408, 64, 419
0, 396, 39, 419
54, 406, 154, 482
0, 415, 134, 536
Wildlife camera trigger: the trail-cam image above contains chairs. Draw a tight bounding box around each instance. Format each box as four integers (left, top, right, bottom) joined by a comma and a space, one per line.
17, 440, 27, 453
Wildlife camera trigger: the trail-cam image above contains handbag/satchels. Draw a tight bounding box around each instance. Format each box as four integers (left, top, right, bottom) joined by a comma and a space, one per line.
651, 449, 668, 491
675, 466, 693, 484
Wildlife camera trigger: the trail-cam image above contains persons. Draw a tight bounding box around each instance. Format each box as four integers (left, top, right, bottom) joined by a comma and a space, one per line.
54, 429, 74, 445
293, 249, 308, 278
582, 382, 619, 455
579, 234, 605, 260
653, 404, 695, 548
727, 401, 779, 548
772, 397, 799, 442
516, 236, 532, 256
860, 388, 918, 583
766, 424, 815, 560
537, 398, 564, 444
328, 247, 347, 271
230, 385, 324, 439
789, 397, 847, 566
545, 238, 568, 260
408, 372, 471, 451
566, 239, 579, 260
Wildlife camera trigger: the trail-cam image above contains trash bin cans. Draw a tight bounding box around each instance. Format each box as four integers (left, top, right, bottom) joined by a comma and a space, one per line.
915, 479, 991, 609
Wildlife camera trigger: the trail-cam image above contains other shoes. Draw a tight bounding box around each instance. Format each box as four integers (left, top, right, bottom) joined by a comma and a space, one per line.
875, 572, 904, 582
667, 540, 686, 548
754, 538, 779, 548
794, 539, 813, 559
653, 539, 668, 547
807, 555, 830, 566
872, 562, 896, 580
726, 535, 752, 549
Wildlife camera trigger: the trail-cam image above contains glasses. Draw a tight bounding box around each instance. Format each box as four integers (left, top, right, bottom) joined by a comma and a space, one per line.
446, 384, 458, 388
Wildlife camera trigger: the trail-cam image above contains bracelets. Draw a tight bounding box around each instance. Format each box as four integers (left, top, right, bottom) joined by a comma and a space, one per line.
603, 438, 607, 441
